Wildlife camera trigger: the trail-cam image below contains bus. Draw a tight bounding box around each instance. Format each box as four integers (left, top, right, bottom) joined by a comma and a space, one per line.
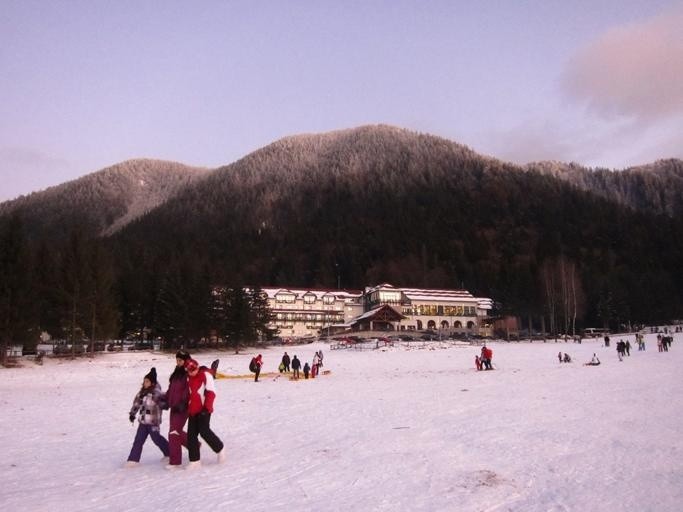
583, 327, 609, 339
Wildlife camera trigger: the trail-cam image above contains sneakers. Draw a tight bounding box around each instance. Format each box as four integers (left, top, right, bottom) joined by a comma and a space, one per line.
183, 461, 201, 471
216, 442, 225, 464
160, 456, 169, 463
165, 464, 183, 470
122, 461, 139, 467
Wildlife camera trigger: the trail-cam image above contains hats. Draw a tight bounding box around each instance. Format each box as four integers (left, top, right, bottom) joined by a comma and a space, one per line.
145, 368, 156, 383
184, 358, 199, 372
176, 349, 190, 360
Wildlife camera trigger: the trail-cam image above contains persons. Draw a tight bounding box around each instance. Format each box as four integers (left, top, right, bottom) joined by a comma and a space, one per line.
474, 355, 482, 370
281, 351, 290, 372
302, 362, 310, 379
278, 362, 285, 372
615, 332, 673, 361
374, 336, 379, 349
120, 367, 170, 469
563, 352, 570, 363
249, 354, 263, 381
182, 358, 225, 470
591, 352, 599, 364
311, 351, 319, 378
557, 351, 562, 362
159, 348, 201, 470
603, 333, 611, 346
291, 354, 302, 380
482, 347, 494, 369
479, 347, 488, 370
317, 349, 323, 368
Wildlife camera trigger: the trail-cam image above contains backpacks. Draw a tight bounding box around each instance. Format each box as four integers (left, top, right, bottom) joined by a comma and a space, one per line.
249, 357, 257, 372
